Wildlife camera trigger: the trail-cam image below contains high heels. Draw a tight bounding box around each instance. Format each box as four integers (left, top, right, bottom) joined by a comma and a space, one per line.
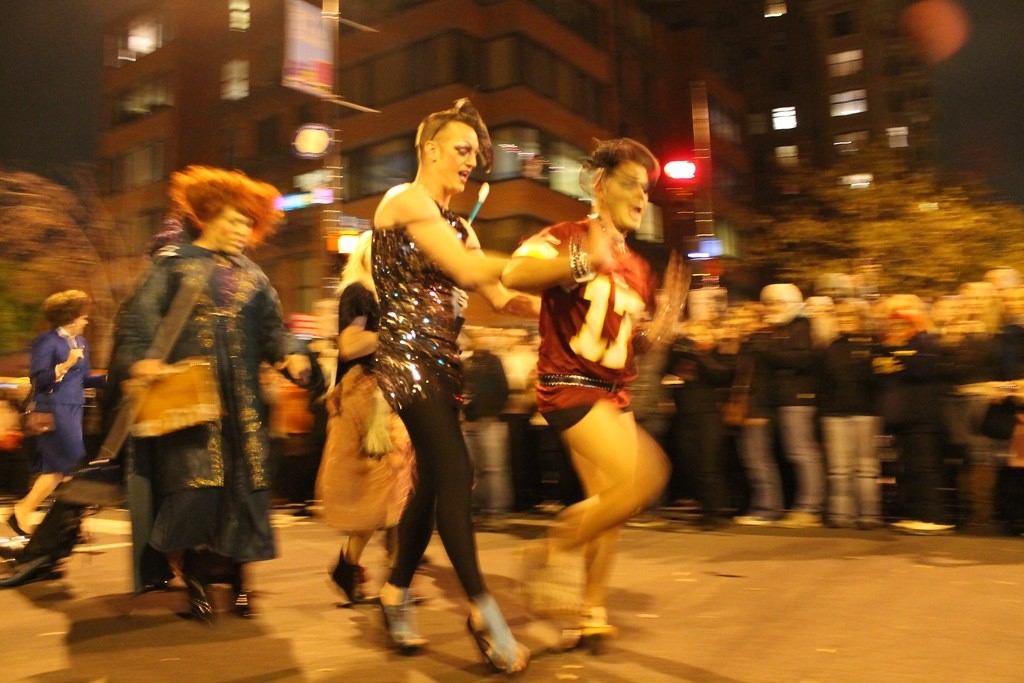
377, 590, 425, 657
465, 615, 529, 675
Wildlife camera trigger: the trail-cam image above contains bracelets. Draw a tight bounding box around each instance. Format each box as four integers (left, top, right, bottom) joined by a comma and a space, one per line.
59, 363, 67, 374
571, 242, 597, 283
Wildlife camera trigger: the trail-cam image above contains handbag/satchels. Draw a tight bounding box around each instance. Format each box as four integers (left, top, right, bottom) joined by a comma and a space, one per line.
23, 380, 55, 433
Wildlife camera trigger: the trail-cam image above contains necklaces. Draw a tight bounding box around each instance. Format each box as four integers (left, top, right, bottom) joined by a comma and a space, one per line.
597, 216, 625, 253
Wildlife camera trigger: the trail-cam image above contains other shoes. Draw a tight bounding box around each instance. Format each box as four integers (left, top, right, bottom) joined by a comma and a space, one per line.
626, 498, 964, 541
186, 580, 213, 620
13, 504, 29, 535
390, 546, 429, 566
518, 556, 549, 618
230, 574, 257, 618
330, 548, 380, 604
575, 606, 620, 641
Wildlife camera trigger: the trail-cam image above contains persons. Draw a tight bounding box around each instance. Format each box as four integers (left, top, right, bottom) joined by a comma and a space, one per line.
314, 97, 530, 673
660, 269, 1024, 535
7, 290, 108, 538
108, 163, 332, 623
501, 139, 693, 655
